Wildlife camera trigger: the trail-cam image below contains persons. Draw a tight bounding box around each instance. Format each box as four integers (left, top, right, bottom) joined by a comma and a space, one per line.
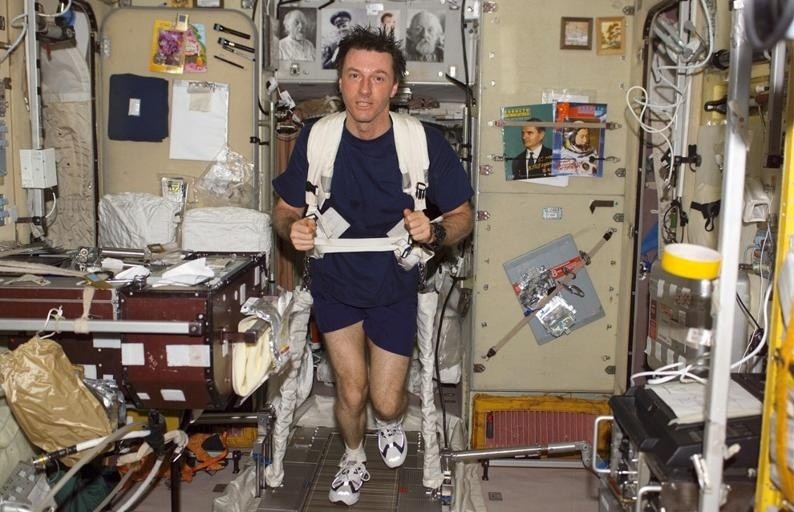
509, 118, 553, 183
270, 25, 475, 509
277, 9, 316, 62
404, 10, 444, 65
553, 118, 602, 174
320, 12, 357, 68
378, 12, 400, 47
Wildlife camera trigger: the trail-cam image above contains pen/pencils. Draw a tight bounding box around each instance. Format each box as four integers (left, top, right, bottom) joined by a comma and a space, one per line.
214, 23, 256, 68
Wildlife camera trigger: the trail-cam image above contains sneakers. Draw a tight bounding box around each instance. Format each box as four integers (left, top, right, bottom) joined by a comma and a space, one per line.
375, 415, 410, 470
327, 451, 373, 507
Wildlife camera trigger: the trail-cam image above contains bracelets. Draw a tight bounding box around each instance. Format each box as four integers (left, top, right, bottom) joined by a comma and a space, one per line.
432, 221, 447, 245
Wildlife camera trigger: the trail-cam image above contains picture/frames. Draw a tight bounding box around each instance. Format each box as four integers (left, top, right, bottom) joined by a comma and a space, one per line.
559, 15, 627, 56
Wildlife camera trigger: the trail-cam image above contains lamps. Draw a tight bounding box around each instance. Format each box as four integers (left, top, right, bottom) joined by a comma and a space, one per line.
660, 243, 770, 280
